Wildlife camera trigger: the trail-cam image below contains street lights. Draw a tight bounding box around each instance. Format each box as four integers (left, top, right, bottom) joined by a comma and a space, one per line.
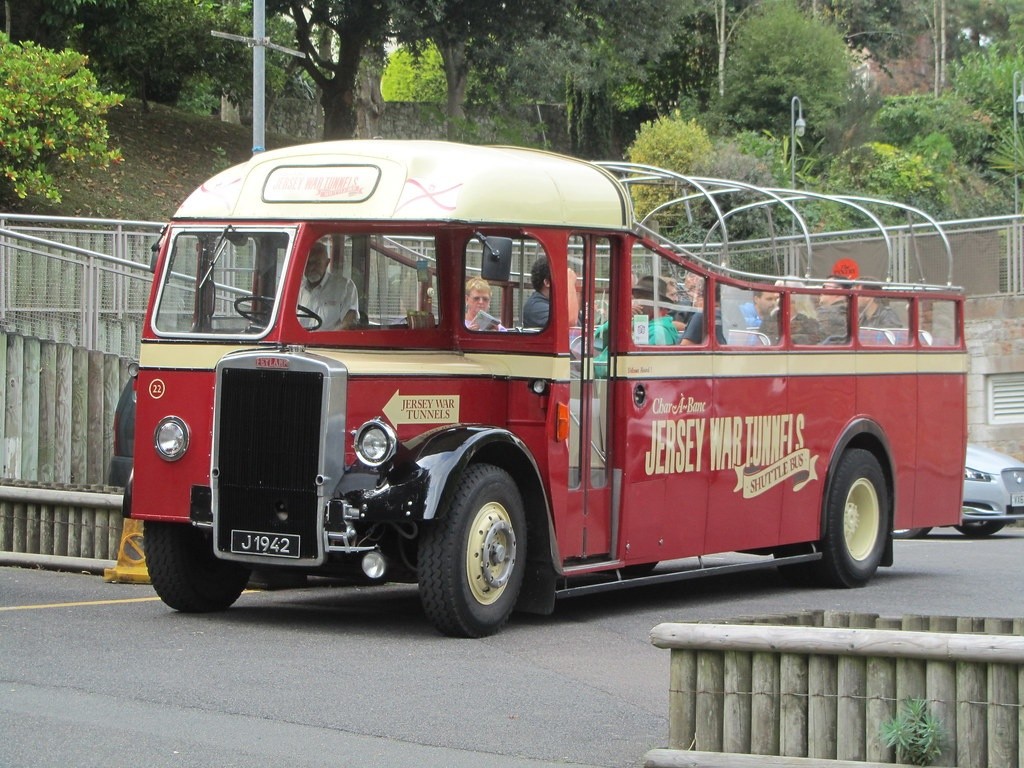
1013, 72, 1023, 296
790, 95, 806, 275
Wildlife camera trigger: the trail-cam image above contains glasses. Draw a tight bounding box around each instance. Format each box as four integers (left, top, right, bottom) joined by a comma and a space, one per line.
469, 293, 489, 302
758, 293, 779, 300
822, 284, 841, 289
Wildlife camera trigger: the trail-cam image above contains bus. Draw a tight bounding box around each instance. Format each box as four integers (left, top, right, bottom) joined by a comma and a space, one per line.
110, 136, 969, 639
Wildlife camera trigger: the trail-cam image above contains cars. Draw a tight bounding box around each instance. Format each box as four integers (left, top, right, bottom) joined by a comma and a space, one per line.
891, 442, 1024, 540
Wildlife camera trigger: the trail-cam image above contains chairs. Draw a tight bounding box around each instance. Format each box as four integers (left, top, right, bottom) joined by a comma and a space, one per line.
888, 328, 932, 346
858, 327, 895, 346
727, 330, 771, 346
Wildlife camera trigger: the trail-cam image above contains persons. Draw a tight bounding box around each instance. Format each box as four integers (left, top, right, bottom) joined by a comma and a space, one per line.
296, 242, 360, 332
593, 269, 903, 376
522, 257, 579, 326
465, 277, 506, 331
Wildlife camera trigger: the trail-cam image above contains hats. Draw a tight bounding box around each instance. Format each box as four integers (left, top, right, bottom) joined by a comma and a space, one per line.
633, 275, 674, 303
